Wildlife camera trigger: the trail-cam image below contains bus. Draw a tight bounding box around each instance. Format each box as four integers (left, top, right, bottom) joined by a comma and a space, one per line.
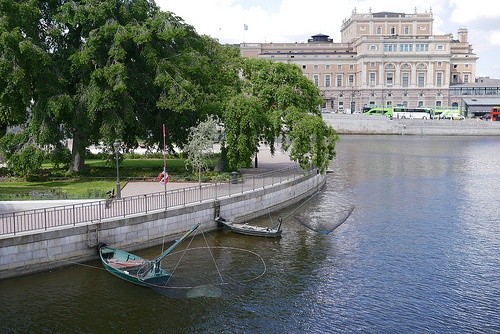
431, 105, 466, 120
393, 107, 431, 120
491, 106, 500, 121
363, 106, 393, 118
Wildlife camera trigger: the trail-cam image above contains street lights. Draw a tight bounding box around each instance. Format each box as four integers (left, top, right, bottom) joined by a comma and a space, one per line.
113, 140, 123, 200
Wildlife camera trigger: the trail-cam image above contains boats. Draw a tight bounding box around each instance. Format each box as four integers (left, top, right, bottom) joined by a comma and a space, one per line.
215, 189, 322, 238
97, 221, 201, 287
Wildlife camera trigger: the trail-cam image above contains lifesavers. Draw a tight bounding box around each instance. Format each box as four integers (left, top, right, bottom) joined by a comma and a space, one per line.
159, 171, 169, 186
